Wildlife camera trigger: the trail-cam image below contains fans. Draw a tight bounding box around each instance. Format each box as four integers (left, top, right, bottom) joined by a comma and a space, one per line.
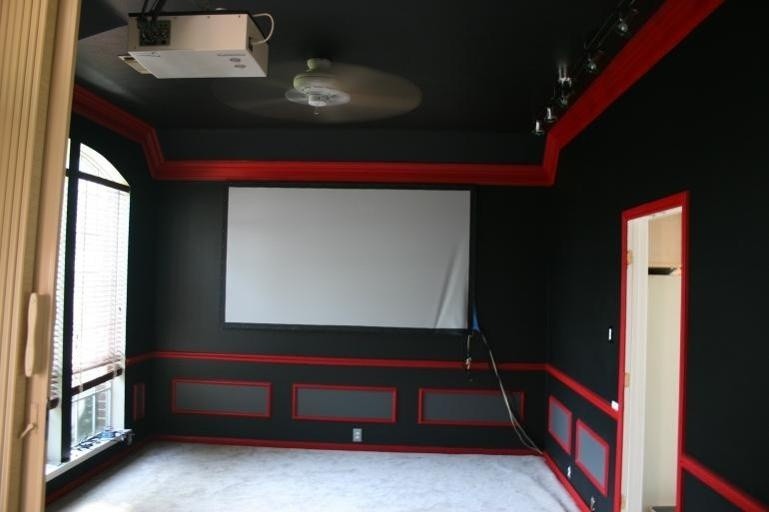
213, 59, 423, 125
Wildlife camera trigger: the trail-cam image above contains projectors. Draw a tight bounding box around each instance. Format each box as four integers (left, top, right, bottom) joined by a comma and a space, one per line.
127, 12, 270, 79
